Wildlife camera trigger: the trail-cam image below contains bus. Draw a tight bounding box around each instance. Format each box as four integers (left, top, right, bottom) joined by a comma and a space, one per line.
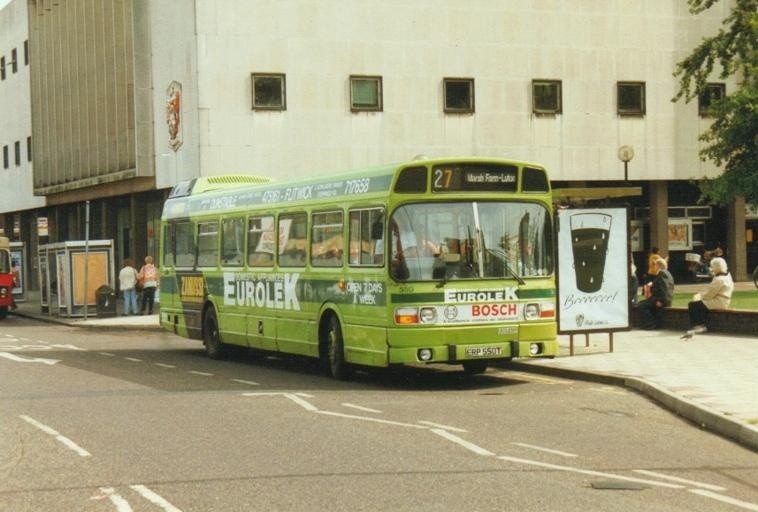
158, 152, 560, 380
0, 235, 13, 320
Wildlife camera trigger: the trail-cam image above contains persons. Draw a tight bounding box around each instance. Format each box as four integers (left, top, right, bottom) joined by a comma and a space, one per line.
647, 248, 670, 280
639, 259, 674, 330
118, 258, 140, 316
679, 257, 735, 337
138, 255, 158, 314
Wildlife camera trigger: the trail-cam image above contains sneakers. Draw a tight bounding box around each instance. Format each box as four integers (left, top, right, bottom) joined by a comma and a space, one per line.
691, 324, 708, 334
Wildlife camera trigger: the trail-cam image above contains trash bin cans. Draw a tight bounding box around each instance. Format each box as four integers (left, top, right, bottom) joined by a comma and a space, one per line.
95, 284, 117, 318
